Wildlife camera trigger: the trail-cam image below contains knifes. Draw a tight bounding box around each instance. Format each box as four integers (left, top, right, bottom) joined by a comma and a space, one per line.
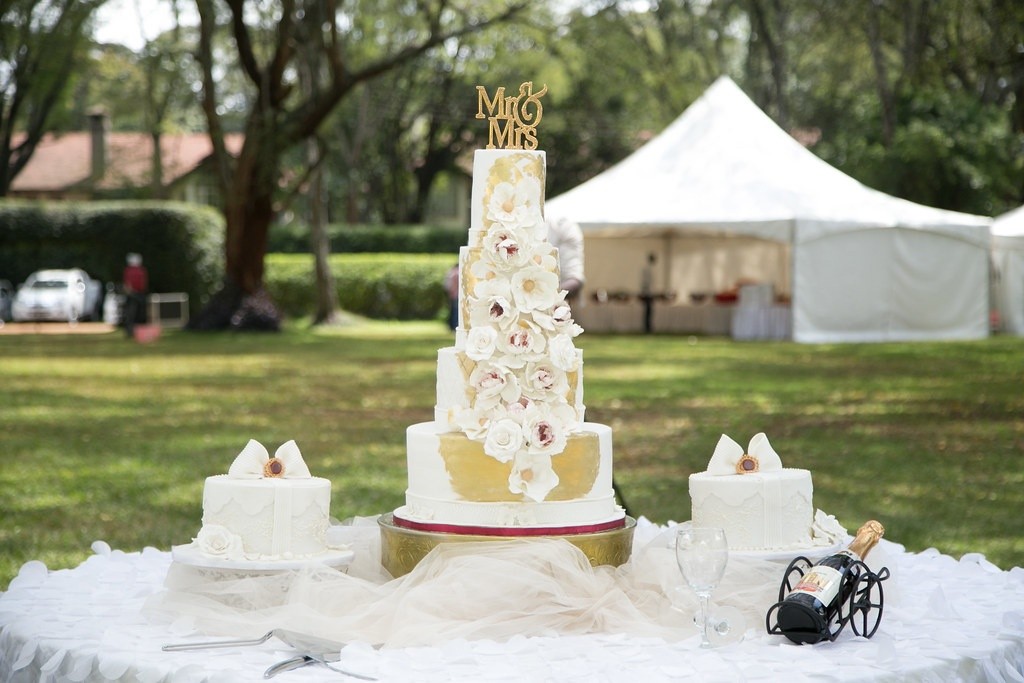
264, 642, 383, 678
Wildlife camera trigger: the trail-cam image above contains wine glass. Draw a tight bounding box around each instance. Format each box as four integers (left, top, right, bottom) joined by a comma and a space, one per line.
675, 530, 729, 651
674, 595, 745, 649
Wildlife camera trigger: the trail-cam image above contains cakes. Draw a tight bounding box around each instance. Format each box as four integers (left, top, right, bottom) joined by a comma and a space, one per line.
185, 439, 333, 559
687, 430, 816, 549
402, 80, 617, 522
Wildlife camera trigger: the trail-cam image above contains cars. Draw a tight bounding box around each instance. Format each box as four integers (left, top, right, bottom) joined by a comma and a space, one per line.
0, 267, 104, 323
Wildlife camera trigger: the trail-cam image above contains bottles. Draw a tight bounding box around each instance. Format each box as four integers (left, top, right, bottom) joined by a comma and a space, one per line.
778, 520, 884, 645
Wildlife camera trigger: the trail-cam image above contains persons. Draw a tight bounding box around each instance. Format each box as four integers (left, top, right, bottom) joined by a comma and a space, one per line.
121, 252, 147, 337
639, 252, 656, 334
547, 216, 585, 309
445, 265, 459, 331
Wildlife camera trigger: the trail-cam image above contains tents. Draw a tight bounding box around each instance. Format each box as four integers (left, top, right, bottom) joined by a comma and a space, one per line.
542, 76, 1024, 345
